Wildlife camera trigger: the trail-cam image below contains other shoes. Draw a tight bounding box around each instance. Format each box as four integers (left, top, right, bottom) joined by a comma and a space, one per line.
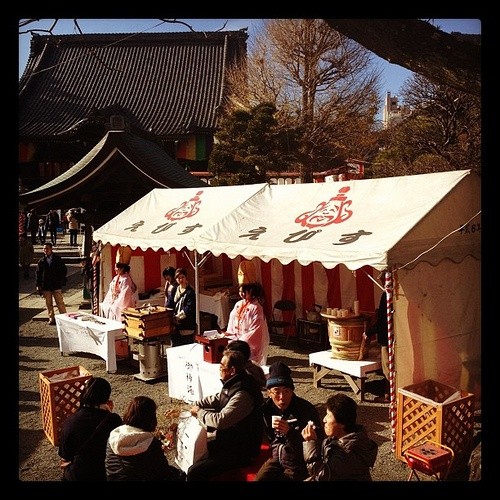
31, 241, 39, 245
48, 321, 56, 325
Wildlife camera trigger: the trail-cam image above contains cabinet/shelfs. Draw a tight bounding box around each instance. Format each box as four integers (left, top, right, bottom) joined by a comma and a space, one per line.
297, 318, 328, 349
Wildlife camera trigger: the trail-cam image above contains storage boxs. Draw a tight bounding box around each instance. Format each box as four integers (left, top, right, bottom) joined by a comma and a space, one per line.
408, 444, 451, 476
199, 274, 224, 286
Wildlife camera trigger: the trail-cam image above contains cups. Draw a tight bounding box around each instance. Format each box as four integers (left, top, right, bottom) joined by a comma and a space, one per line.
326, 307, 348, 318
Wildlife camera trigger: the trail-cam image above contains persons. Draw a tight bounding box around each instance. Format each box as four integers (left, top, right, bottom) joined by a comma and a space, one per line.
164, 267, 197, 348
254, 360, 322, 484
99, 245, 138, 332
222, 340, 266, 404
26, 201, 86, 247
162, 265, 178, 320
103, 395, 182, 484
214, 259, 270, 369
34, 242, 68, 326
56, 375, 125, 486
300, 393, 378, 482
185, 352, 268, 483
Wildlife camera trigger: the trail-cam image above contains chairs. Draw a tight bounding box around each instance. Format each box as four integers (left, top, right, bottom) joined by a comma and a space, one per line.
268, 300, 297, 347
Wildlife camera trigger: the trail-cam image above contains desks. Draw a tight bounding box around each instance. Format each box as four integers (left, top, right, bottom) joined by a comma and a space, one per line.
139, 298, 165, 307
54, 312, 127, 374
166, 343, 270, 405
309, 349, 382, 401
199, 293, 240, 330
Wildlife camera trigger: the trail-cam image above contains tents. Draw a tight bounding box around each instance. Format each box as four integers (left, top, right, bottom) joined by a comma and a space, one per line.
91, 166, 484, 456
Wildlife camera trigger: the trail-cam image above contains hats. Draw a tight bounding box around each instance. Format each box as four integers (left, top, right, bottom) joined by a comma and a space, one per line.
39, 219, 44, 224
266, 363, 296, 391
